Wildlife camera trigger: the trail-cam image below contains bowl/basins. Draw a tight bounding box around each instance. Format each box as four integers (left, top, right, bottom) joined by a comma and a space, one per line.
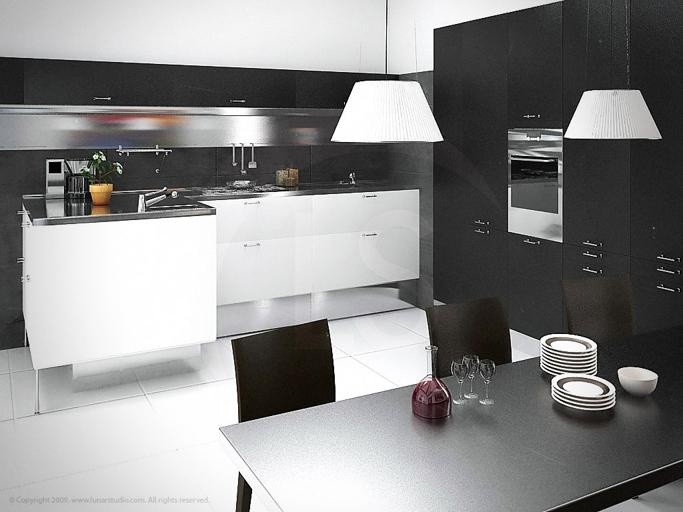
617, 366, 659, 397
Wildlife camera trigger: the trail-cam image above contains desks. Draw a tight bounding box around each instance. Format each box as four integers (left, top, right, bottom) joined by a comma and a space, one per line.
218, 324, 683, 512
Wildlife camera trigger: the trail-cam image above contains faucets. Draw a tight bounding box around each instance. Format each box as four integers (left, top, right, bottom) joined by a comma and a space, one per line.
137, 191, 179, 209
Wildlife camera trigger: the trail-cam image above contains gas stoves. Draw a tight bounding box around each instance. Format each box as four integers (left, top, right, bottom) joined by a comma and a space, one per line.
206, 186, 284, 194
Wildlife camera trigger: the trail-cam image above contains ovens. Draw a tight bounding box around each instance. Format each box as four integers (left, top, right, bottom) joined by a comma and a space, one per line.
505, 129, 562, 242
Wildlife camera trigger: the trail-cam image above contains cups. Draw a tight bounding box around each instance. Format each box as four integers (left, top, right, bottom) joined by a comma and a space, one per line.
63, 197, 92, 216
66, 175, 95, 199
274, 167, 298, 188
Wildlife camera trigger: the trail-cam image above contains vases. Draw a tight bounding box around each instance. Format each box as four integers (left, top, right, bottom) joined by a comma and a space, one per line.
89, 183, 114, 206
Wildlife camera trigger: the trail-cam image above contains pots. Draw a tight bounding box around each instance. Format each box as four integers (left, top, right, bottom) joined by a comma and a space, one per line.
226, 179, 258, 189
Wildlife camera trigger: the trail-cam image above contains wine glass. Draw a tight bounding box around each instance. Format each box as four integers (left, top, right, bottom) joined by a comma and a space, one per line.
478, 359, 495, 405
450, 359, 470, 404
462, 354, 479, 400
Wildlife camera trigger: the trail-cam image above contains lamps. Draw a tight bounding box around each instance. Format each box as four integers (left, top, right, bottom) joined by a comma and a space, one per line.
563, 1, 664, 141
330, 1, 445, 144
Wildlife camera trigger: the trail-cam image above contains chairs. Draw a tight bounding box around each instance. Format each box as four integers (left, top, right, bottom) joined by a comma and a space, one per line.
230, 317, 337, 512
560, 277, 634, 349
425, 295, 512, 382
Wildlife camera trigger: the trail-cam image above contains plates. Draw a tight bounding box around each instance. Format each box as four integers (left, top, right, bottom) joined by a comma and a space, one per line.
539, 333, 597, 380
548, 372, 615, 413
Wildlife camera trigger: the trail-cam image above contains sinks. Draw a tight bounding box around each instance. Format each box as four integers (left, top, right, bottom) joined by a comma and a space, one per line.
151, 205, 198, 209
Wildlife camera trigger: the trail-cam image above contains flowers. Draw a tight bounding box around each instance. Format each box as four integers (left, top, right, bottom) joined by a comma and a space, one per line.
79, 150, 123, 186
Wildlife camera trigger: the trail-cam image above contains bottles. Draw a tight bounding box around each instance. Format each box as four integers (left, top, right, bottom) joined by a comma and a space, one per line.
410, 345, 451, 418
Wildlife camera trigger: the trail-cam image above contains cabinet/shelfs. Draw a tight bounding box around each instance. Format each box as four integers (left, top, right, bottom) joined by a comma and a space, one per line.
433, 0, 681, 340
197, 195, 311, 306
311, 189, 420, 292
